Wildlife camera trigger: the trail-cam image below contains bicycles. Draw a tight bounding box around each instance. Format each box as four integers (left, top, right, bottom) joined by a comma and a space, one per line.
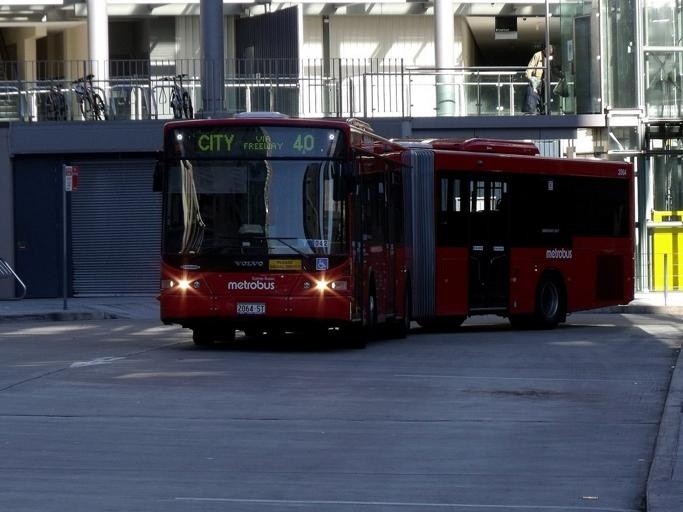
70, 73, 111, 124
41, 74, 74, 122
155, 72, 194, 121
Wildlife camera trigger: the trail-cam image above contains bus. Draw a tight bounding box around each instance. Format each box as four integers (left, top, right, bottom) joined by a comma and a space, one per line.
149, 111, 637, 347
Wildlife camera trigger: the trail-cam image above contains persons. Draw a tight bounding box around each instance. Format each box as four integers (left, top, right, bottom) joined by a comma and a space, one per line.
521, 44, 553, 115
